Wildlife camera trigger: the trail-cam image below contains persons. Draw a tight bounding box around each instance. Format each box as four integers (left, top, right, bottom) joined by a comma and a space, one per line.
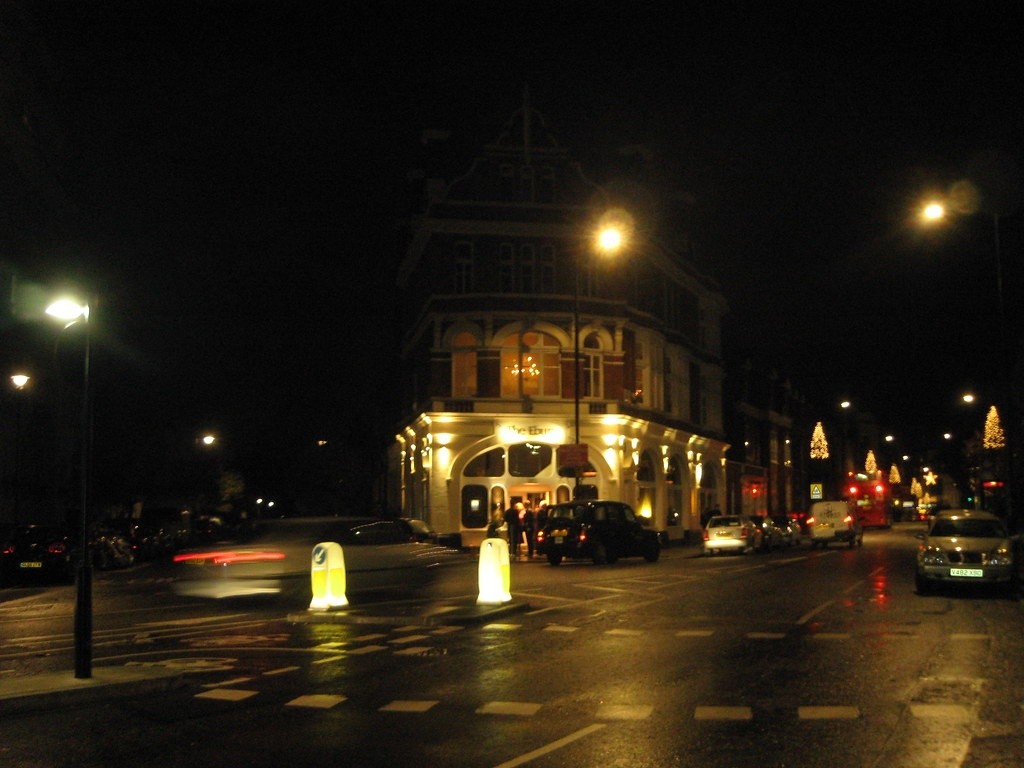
504, 499, 524, 557
989, 490, 1001, 506
522, 499, 535, 556
536, 500, 548, 555
700, 504, 722, 529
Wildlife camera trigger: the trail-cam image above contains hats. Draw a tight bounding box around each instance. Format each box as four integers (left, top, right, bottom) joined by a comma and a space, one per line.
540, 499, 547, 506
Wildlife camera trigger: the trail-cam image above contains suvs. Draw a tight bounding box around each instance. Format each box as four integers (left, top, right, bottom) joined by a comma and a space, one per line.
538, 499, 660, 566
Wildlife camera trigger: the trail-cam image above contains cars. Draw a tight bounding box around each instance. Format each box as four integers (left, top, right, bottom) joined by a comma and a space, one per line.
914, 508, 1024, 592
894, 492, 953, 522
0, 519, 226, 590
750, 515, 801, 547
704, 514, 756, 556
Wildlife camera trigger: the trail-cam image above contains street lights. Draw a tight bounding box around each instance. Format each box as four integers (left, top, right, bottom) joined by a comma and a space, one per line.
575, 221, 624, 501
918, 194, 1019, 537
44, 298, 92, 679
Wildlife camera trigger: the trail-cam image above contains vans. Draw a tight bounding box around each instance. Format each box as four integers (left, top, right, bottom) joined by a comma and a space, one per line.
807, 500, 865, 548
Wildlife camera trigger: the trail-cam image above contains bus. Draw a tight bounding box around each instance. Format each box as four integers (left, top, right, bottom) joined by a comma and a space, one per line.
845, 479, 895, 531
